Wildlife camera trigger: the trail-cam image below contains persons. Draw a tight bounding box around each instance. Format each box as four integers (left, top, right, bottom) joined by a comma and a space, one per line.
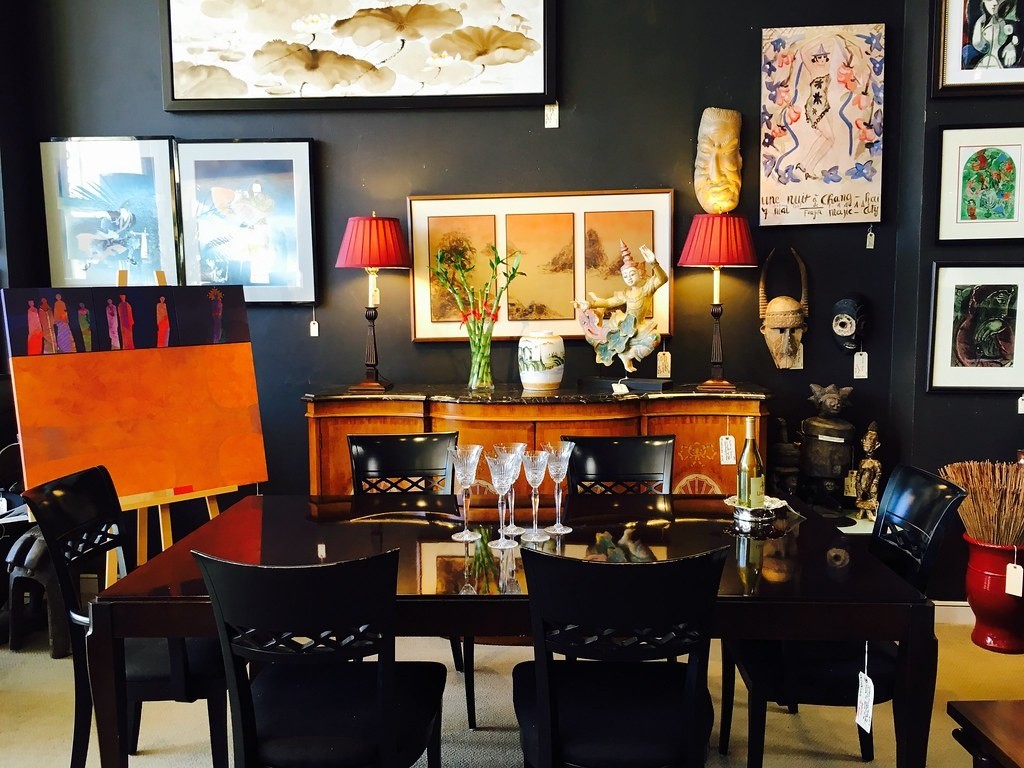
589, 236, 668, 371
853, 422, 882, 520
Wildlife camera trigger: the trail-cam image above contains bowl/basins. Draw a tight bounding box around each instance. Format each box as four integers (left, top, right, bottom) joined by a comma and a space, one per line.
723, 495, 788, 523
724, 521, 788, 541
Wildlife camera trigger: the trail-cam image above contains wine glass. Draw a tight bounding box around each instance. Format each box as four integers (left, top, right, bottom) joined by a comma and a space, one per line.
454, 536, 564, 596
446, 444, 484, 542
521, 449, 551, 543
540, 441, 575, 535
487, 454, 519, 550
493, 442, 526, 535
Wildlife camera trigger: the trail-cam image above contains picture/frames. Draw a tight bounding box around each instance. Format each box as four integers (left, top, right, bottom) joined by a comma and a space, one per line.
414, 539, 673, 595
933, 122, 1024, 247
156, 0, 558, 114
407, 189, 677, 345
175, 136, 324, 308
34, 132, 181, 289
929, 0, 1024, 101
924, 260, 1024, 395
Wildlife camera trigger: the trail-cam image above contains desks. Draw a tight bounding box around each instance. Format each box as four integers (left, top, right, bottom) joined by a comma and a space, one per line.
85, 493, 940, 768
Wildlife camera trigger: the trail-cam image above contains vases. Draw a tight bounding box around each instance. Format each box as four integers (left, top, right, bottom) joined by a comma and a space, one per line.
518, 330, 565, 391
961, 531, 1024, 655
464, 319, 495, 393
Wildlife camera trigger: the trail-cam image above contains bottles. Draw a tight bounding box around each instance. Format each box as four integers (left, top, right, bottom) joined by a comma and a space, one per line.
736, 535, 763, 598
736, 417, 765, 508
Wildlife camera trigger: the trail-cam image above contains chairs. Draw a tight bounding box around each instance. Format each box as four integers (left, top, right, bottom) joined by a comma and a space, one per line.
511, 545, 732, 768
189, 546, 447, 768
19, 464, 248, 768
345, 431, 464, 674
559, 434, 678, 662
717, 462, 966, 768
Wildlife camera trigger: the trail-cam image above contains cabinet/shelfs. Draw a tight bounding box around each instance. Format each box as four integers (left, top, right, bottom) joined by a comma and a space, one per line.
300, 380, 774, 521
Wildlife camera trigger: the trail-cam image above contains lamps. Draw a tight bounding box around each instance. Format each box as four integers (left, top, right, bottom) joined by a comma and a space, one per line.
676, 213, 758, 395
334, 208, 414, 391
